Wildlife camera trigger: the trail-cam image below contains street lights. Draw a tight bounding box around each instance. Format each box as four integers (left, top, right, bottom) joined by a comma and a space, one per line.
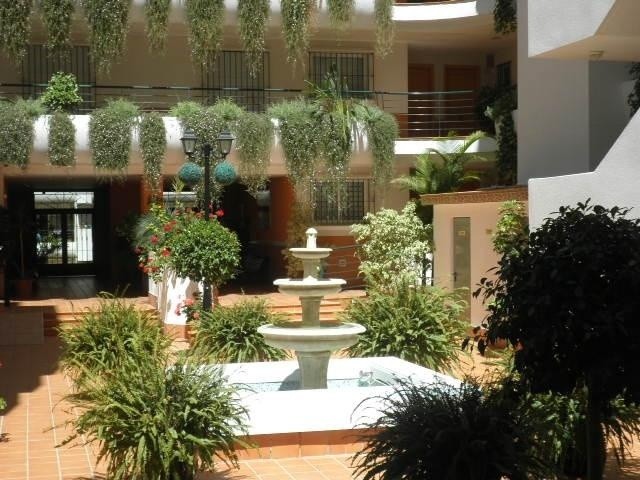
178, 122, 234, 317
